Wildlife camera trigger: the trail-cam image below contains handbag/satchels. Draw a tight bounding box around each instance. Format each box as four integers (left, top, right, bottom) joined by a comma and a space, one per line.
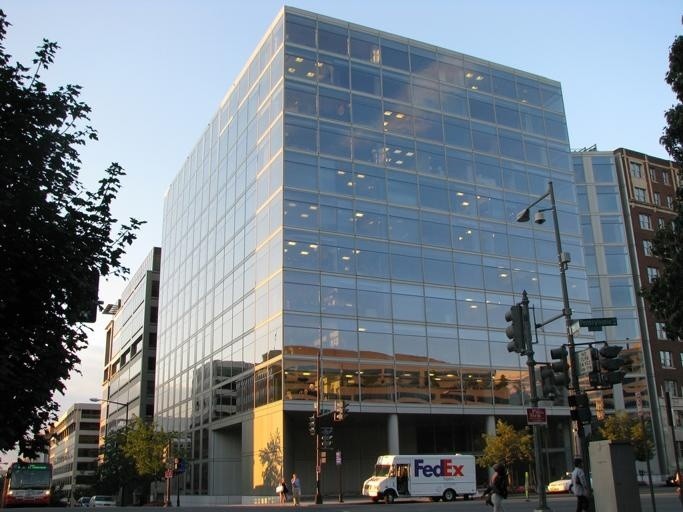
575, 468, 581, 484
275, 485, 284, 494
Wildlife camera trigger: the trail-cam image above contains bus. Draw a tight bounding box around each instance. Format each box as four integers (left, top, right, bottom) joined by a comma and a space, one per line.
0, 460, 52, 506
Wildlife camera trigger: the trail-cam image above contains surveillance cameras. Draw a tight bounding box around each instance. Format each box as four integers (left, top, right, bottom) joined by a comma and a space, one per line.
535, 212, 546, 224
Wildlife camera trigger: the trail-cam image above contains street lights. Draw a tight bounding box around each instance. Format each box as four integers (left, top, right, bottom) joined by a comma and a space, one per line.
510, 170, 596, 511
88, 396, 129, 506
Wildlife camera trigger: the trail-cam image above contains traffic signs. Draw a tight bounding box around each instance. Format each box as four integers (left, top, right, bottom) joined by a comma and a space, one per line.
574, 347, 592, 377
570, 317, 618, 328
569, 319, 579, 334
164, 469, 173, 479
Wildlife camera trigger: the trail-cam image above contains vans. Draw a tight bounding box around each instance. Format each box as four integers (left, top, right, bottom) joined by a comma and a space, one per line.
60, 494, 118, 508
361, 453, 477, 504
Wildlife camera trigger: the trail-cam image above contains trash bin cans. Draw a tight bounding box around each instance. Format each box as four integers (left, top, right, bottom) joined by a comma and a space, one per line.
132, 488, 142, 505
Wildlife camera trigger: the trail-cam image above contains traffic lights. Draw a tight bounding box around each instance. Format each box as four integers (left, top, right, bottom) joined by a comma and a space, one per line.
550, 346, 570, 385
599, 345, 625, 385
586, 348, 603, 387
318, 427, 326, 449
173, 457, 179, 469
161, 446, 167, 463
333, 399, 345, 422
504, 291, 534, 355
306, 412, 318, 436
538, 363, 557, 398
567, 392, 590, 422
325, 427, 335, 450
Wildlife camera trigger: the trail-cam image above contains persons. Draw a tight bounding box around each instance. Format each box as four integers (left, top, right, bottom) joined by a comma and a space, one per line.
479, 463, 508, 512
569, 457, 589, 512
278, 479, 284, 504
282, 478, 289, 502
290, 473, 301, 507
396, 466, 407, 489
486, 462, 495, 483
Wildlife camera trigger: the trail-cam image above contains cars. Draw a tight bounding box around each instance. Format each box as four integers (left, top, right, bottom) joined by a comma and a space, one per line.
546, 471, 593, 494
